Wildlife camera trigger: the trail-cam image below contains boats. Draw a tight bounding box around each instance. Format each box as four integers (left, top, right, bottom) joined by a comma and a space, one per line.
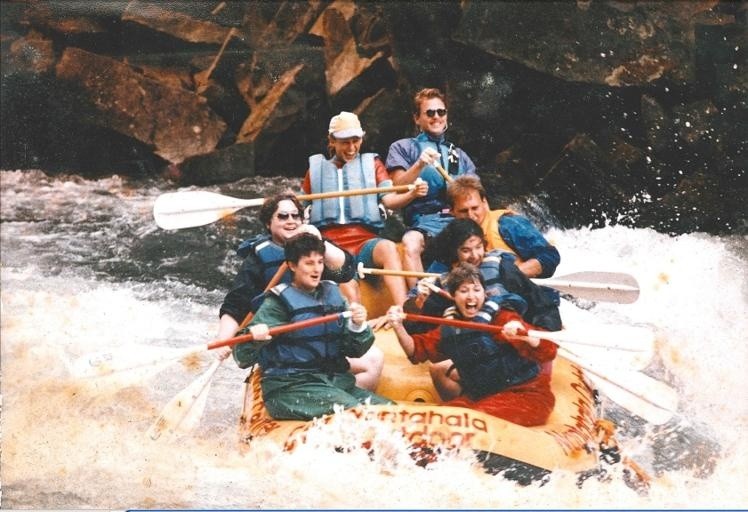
234, 241, 602, 492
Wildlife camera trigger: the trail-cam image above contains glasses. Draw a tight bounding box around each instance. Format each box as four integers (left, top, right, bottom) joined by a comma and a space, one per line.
426, 109, 445, 117
277, 212, 300, 219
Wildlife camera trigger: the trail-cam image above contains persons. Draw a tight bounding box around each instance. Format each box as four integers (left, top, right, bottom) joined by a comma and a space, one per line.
402, 217, 563, 334
385, 87, 480, 292
385, 260, 557, 426
297, 111, 430, 305
231, 233, 397, 421
408, 176, 560, 301
215, 193, 384, 392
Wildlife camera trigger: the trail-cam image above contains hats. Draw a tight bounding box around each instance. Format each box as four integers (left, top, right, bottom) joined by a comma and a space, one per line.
329, 111, 366, 138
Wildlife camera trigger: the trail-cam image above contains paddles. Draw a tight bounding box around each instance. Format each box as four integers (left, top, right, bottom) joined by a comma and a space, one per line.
154, 177, 418, 230
396, 307, 655, 370
556, 346, 679, 426
357, 261, 639, 304
74, 309, 350, 397
156, 261, 289, 434
